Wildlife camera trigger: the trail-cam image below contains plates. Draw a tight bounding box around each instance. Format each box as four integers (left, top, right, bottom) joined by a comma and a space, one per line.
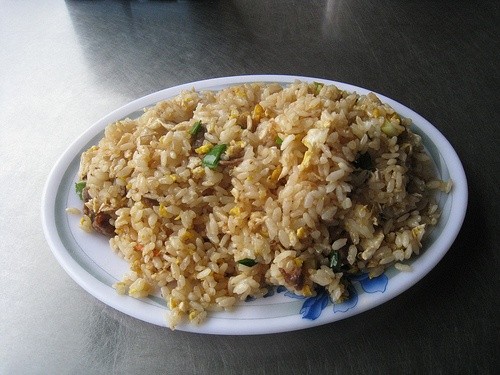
41, 75, 468, 334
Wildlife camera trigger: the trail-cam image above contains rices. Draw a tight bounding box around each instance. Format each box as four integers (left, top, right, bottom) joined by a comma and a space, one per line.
65, 78, 453, 331
